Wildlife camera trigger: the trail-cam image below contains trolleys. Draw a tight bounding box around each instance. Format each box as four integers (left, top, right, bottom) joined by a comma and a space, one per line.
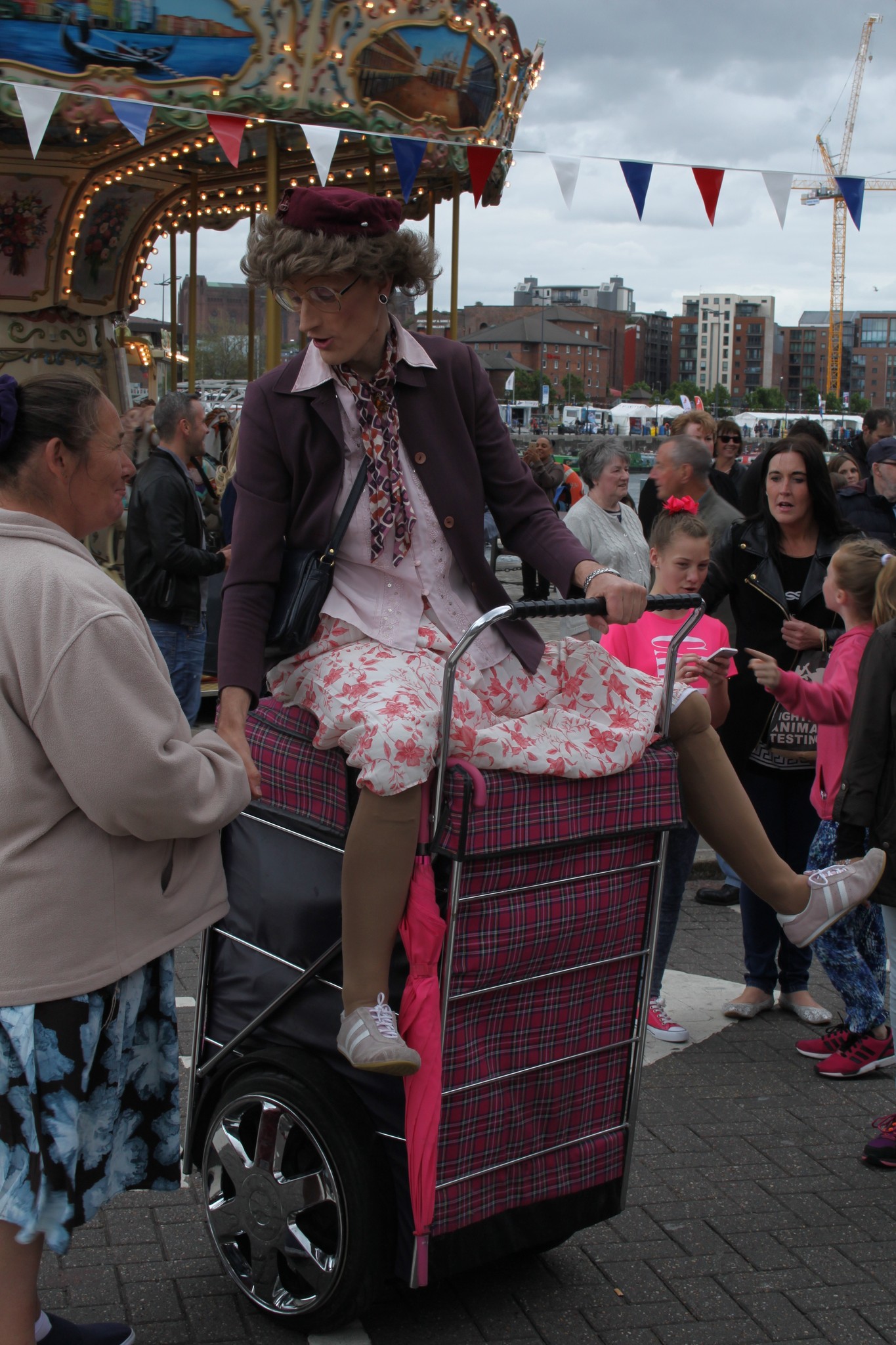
181, 591, 707, 1330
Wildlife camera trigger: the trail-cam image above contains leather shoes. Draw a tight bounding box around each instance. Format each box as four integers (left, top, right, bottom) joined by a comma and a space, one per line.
694, 883, 742, 904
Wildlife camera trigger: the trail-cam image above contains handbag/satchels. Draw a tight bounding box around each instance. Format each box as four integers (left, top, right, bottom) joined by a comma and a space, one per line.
265, 548, 336, 648
200, 491, 222, 542
769, 627, 829, 754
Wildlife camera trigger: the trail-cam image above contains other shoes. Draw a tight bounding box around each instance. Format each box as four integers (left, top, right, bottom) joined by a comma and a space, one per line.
778, 994, 833, 1023
721, 995, 774, 1018
530, 593, 548, 601
517, 594, 532, 601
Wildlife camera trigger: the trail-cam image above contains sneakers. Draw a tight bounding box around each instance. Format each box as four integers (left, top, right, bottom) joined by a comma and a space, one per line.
813, 1025, 896, 1078
36, 1311, 135, 1345
776, 847, 885, 947
860, 1113, 896, 1169
795, 1011, 852, 1059
336, 992, 421, 1076
634, 998, 688, 1043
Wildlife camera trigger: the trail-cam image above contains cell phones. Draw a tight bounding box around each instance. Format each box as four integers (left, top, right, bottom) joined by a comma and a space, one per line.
529, 442, 537, 452
705, 648, 739, 664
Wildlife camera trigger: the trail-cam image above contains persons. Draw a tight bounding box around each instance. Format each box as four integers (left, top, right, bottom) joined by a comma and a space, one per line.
0, 373, 250, 1345
123, 389, 242, 725
484, 411, 896, 1167
215, 187, 886, 1077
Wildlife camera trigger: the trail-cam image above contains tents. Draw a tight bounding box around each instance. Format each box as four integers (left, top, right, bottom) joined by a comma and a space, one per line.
610, 403, 863, 440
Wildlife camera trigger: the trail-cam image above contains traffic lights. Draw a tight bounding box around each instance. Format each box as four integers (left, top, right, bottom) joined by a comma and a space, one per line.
710, 403, 715, 415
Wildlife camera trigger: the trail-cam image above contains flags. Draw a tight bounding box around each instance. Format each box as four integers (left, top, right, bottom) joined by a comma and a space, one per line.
504, 371, 514, 391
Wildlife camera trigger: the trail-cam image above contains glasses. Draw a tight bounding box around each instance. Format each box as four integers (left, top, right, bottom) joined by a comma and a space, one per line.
271, 274, 364, 314
717, 436, 741, 443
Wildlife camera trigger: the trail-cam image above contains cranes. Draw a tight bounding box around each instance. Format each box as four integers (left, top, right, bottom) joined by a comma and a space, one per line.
789, 11, 896, 409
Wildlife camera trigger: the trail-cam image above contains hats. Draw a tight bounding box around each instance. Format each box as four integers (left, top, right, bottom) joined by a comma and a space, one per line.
275, 186, 402, 238
866, 437, 896, 467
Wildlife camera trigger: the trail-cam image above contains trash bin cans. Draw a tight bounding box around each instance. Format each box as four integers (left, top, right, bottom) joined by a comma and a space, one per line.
609, 429, 614, 434
651, 427, 658, 436
660, 425, 664, 435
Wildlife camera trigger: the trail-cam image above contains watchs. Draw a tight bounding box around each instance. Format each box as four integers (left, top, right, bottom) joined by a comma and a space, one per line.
584, 568, 621, 594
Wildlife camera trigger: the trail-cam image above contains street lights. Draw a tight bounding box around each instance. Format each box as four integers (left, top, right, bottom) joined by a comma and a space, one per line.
655, 396, 660, 436
567, 360, 572, 405
799, 392, 802, 414
724, 399, 729, 420
870, 393, 874, 407
834, 319, 863, 414
585, 393, 592, 435
523, 288, 553, 429
779, 377, 784, 413
750, 391, 754, 412
504, 390, 511, 423
154, 274, 182, 330
883, 353, 889, 408
699, 304, 730, 418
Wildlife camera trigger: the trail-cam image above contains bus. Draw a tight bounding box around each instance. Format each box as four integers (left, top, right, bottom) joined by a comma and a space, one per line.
562, 405, 612, 435
